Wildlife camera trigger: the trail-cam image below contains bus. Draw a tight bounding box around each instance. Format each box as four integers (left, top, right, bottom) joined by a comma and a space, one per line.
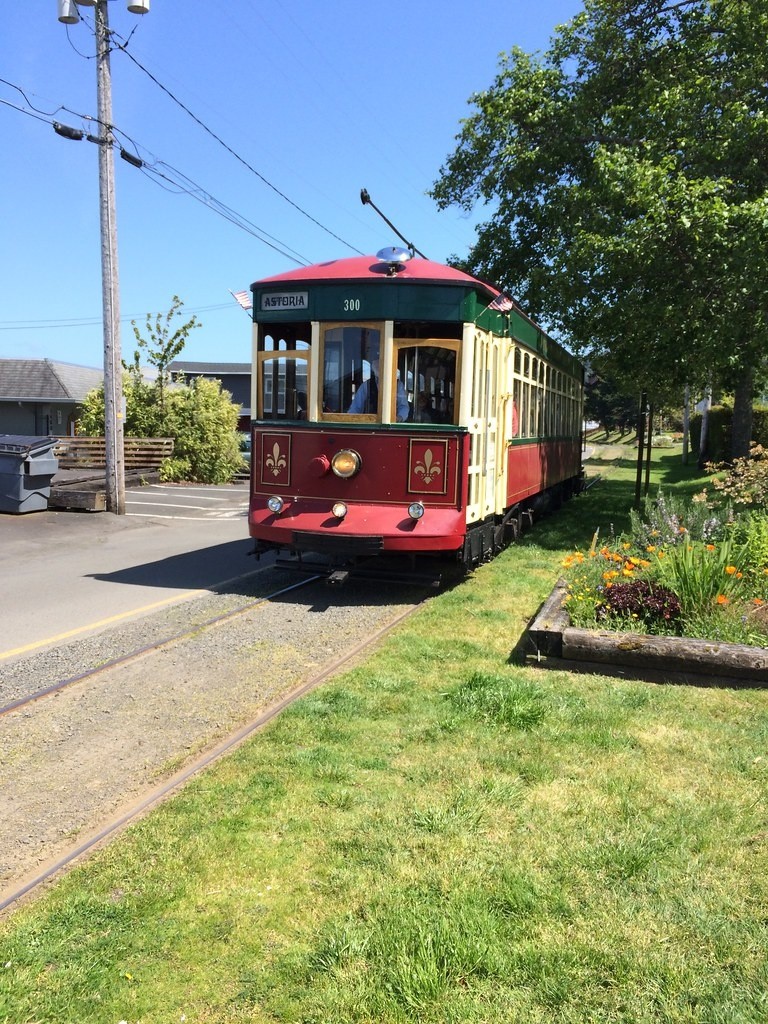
250, 246, 586, 588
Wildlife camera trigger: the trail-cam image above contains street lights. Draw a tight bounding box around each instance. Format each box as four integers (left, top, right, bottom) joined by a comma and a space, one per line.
59, 0, 150, 516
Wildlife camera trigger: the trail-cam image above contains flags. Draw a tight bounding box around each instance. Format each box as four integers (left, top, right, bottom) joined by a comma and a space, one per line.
234, 290, 253, 310
488, 295, 514, 312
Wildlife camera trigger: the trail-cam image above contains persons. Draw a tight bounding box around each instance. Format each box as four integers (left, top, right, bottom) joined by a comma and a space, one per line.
347, 348, 410, 422
411, 391, 432, 423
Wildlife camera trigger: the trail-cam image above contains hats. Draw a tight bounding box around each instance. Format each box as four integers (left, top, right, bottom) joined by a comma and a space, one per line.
366, 344, 380, 360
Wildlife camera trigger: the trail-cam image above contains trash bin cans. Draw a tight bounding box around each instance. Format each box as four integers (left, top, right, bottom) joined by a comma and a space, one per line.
0, 433, 61, 513
